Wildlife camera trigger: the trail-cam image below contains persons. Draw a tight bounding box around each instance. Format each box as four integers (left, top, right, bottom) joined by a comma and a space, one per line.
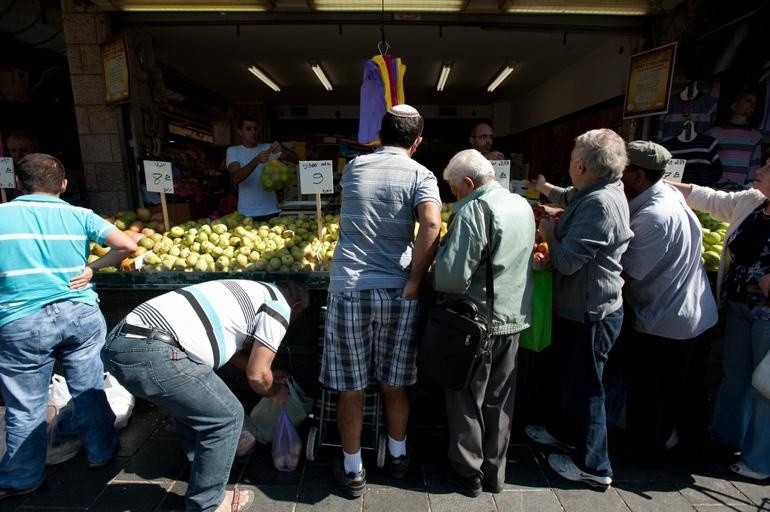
0, 153, 137, 498
102, 272, 310, 511
671, 138, 768, 481
621, 138, 722, 476
708, 86, 765, 183
424, 149, 537, 494
511, 130, 626, 492
224, 112, 300, 219
325, 103, 448, 496
469, 118, 505, 164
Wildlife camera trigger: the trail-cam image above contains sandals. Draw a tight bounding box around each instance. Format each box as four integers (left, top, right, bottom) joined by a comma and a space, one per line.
730, 461, 768, 480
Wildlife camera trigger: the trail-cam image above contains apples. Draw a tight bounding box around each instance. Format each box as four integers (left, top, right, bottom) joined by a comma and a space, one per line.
88, 208, 339, 272
533, 229, 548, 262
694, 213, 730, 272
441, 203, 453, 237
260, 166, 291, 192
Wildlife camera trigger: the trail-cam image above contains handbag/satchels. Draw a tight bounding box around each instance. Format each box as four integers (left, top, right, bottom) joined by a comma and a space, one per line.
414, 272, 491, 392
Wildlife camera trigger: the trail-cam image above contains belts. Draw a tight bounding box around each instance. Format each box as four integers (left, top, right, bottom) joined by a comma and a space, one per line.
116, 323, 181, 347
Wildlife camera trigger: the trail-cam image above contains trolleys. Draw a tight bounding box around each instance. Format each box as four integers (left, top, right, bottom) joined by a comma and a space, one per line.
298, 301, 390, 473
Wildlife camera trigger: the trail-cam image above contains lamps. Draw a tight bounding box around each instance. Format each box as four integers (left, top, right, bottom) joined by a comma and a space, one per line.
311, 64, 334, 92
247, 64, 281, 93
486, 65, 515, 93
437, 63, 451, 92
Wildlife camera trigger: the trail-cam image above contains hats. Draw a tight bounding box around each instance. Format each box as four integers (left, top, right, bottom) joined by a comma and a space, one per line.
627, 139, 673, 172
388, 103, 422, 118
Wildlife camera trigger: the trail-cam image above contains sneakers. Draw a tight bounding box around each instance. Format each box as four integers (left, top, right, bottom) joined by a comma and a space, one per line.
215, 488, 255, 512
392, 455, 411, 477
525, 422, 582, 452
546, 449, 612, 493
333, 454, 368, 497
